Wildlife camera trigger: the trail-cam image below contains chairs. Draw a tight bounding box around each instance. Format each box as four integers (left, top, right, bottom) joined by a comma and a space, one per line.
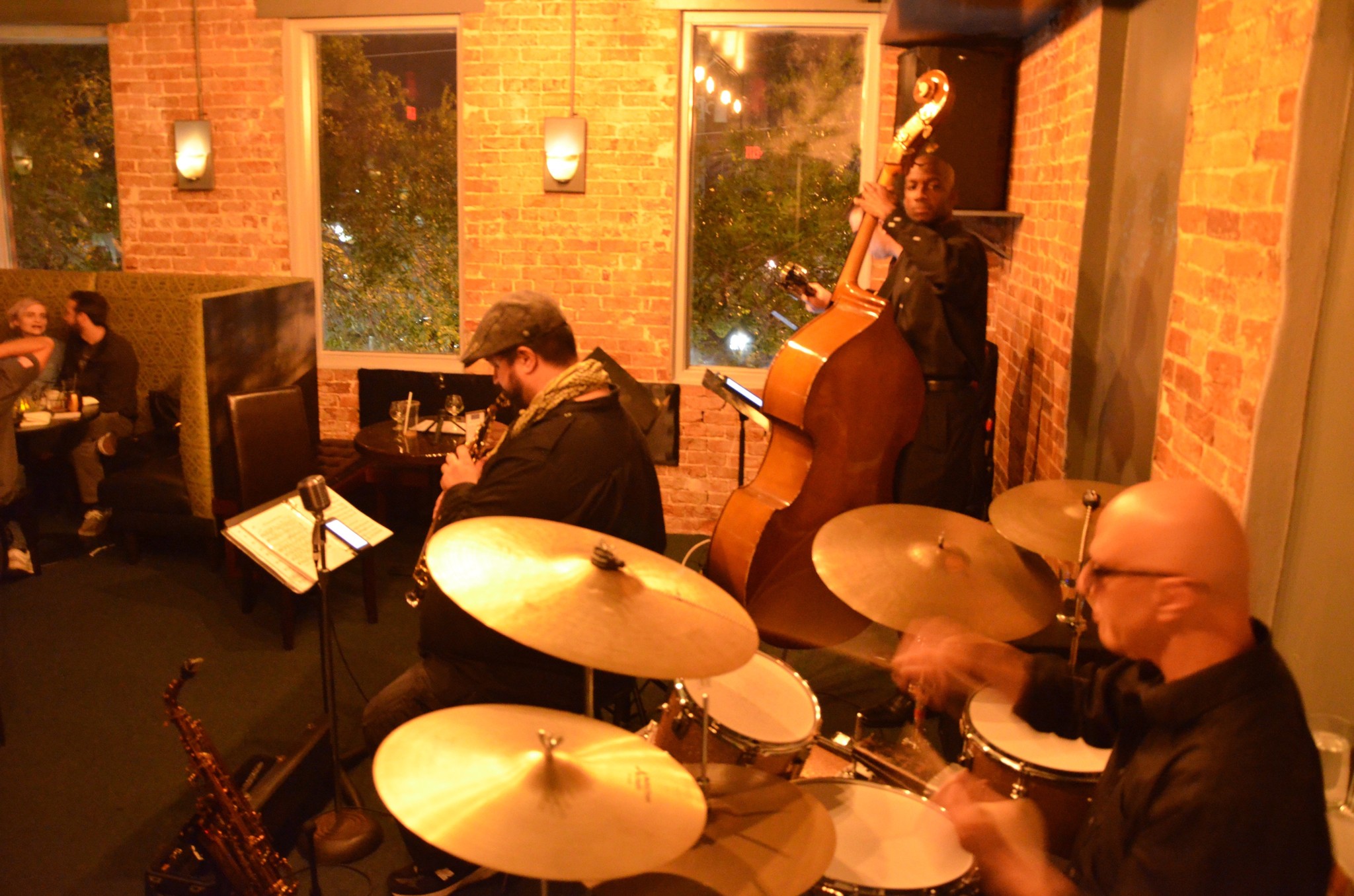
226, 385, 376, 648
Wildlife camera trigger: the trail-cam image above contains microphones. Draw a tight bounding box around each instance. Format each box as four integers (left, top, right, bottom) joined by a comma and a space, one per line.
298, 474, 331, 566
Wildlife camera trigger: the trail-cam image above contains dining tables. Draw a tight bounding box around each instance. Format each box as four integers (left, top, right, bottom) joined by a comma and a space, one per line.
355, 422, 473, 622
13, 388, 98, 533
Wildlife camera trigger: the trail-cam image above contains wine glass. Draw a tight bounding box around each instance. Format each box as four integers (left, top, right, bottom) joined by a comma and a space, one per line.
444, 394, 465, 433
389, 401, 406, 431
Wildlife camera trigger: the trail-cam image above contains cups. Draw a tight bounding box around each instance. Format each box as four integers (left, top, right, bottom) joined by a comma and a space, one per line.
399, 400, 420, 438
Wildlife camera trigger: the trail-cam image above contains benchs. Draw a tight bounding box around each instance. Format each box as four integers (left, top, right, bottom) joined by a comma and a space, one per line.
211, 437, 366, 583
32, 438, 196, 541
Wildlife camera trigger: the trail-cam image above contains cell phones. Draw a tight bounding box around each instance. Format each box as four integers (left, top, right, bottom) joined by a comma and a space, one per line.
324, 517, 371, 552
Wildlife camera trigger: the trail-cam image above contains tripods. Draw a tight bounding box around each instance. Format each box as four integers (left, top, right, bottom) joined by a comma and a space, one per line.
423, 374, 466, 445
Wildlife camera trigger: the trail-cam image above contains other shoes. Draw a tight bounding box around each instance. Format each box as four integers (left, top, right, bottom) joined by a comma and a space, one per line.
6, 547, 33, 573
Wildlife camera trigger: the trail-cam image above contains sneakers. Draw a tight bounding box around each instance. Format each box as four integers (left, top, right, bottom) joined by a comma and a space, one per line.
96, 432, 117, 455
78, 507, 111, 535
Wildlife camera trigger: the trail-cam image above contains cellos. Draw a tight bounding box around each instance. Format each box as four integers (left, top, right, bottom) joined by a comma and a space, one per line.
703, 75, 961, 660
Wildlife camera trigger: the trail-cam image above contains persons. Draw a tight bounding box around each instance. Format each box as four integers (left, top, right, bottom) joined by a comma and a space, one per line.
359, 290, 669, 896
887, 479, 1333, 896
0, 289, 139, 576
801, 156, 987, 523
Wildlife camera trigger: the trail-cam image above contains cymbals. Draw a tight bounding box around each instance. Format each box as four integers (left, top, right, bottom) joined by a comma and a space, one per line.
580, 756, 837, 894
425, 516, 761, 682
370, 700, 706, 882
810, 500, 1063, 643
988, 477, 1140, 566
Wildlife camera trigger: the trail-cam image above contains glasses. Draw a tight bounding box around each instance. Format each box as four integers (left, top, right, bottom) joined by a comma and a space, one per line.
1083, 558, 1209, 594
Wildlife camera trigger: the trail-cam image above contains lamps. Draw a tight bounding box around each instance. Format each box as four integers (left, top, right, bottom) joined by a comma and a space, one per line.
543, 116, 588, 193
173, 119, 214, 189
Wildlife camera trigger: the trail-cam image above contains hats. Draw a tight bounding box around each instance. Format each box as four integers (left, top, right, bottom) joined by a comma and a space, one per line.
460, 291, 565, 368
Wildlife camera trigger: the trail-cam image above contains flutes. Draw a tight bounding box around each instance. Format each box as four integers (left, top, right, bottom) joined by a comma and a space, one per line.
403, 391, 511, 610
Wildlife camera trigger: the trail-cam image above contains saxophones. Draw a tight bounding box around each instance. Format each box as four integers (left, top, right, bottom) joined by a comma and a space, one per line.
146, 655, 303, 896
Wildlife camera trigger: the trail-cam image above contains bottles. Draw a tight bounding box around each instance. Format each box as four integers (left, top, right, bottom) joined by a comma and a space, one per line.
67, 390, 79, 412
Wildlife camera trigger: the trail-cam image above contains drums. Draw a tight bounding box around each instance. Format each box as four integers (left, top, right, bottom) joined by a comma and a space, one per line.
790, 775, 980, 896
648, 649, 822, 787
959, 682, 1110, 850
